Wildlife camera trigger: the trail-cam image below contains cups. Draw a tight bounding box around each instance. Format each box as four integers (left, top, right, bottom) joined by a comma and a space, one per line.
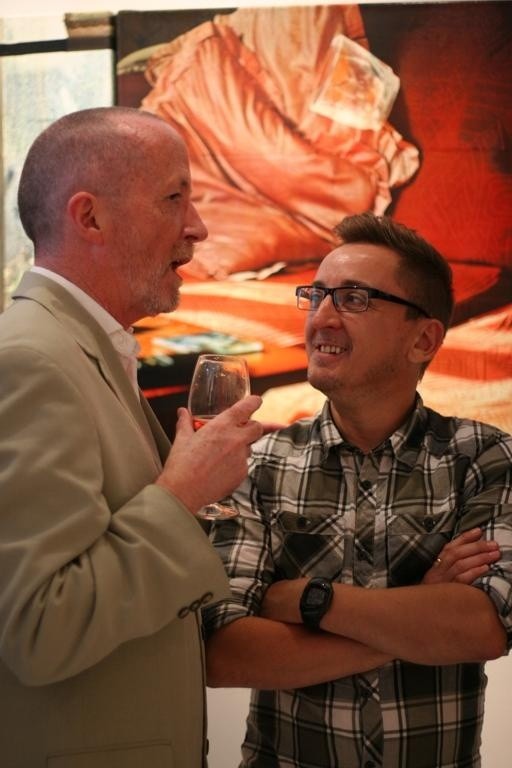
307, 31, 402, 131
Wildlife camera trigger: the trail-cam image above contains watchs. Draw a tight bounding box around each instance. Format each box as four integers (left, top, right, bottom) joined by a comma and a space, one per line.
300, 574, 334, 635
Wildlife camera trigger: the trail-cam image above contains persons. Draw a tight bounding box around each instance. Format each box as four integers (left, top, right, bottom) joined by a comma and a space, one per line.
1, 103, 260, 768
206, 212, 512, 768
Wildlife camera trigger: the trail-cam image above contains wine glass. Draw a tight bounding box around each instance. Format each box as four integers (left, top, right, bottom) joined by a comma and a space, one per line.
188, 355, 252, 522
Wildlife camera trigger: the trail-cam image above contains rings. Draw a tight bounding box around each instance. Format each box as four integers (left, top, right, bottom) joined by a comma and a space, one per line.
436, 557, 442, 563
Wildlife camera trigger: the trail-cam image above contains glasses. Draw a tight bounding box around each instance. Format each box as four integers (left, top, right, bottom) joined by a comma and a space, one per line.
295, 285, 431, 313
